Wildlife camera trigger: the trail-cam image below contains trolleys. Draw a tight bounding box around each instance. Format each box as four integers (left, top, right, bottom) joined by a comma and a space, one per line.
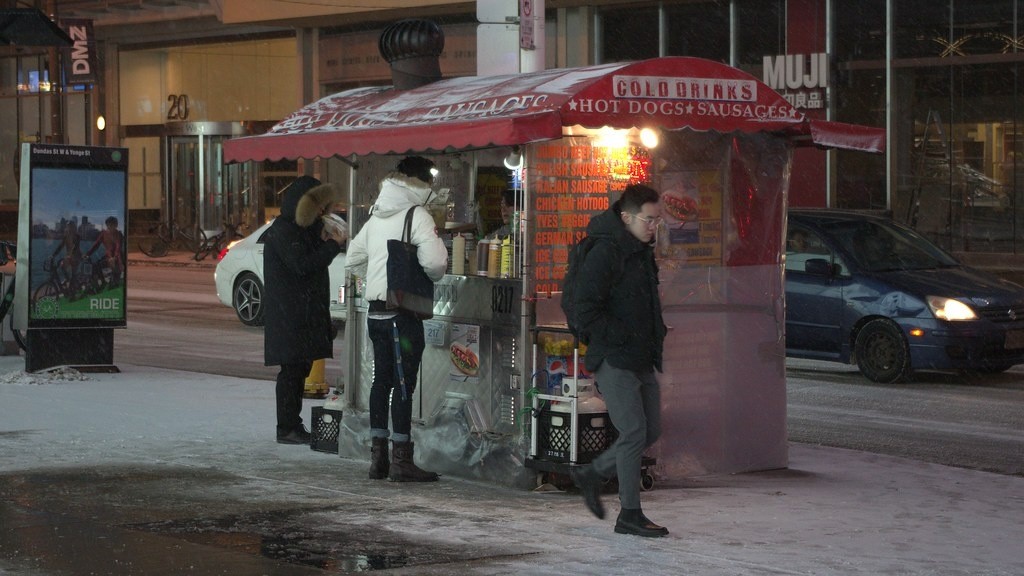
524, 309, 658, 494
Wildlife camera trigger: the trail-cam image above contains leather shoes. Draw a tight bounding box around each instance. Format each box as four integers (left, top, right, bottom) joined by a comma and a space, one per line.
571, 468, 605, 519
615, 509, 670, 536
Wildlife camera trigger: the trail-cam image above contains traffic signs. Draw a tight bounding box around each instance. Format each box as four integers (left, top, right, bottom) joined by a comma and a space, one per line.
518, 0, 535, 50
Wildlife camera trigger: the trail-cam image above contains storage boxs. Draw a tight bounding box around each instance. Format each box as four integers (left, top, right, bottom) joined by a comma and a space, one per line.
542, 356, 601, 403
309, 405, 343, 455
537, 410, 616, 461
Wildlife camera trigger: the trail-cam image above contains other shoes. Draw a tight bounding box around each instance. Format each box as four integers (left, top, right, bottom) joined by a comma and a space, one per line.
278, 423, 310, 444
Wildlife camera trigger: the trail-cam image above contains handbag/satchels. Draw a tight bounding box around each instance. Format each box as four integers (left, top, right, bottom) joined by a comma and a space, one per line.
386, 205, 435, 319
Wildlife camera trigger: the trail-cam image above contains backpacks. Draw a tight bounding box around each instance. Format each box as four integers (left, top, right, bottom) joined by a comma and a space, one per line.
561, 235, 620, 347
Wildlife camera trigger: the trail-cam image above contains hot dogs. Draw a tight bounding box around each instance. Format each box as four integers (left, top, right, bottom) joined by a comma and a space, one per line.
662, 190, 697, 221
450, 340, 479, 375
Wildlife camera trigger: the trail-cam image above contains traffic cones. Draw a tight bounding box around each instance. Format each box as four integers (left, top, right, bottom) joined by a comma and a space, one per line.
303, 359, 331, 400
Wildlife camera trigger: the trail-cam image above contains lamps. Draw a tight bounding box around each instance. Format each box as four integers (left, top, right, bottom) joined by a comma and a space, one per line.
638, 126, 664, 148
503, 147, 524, 169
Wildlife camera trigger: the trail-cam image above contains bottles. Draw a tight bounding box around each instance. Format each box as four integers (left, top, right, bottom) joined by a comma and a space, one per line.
477, 235, 490, 278
452, 232, 465, 275
488, 234, 502, 279
501, 234, 513, 279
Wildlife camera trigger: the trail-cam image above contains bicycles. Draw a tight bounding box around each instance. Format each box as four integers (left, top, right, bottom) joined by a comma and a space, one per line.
192, 217, 251, 262
137, 216, 208, 258
32, 253, 124, 314
0, 239, 27, 352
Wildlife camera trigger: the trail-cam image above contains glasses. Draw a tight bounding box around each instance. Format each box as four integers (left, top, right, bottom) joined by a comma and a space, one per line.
621, 212, 663, 227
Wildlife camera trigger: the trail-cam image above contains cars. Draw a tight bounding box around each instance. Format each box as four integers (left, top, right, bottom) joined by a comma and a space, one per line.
785, 206, 1023, 385
212, 208, 347, 325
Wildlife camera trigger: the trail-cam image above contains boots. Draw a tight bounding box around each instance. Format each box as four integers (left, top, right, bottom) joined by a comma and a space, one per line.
369, 438, 392, 479
389, 440, 439, 481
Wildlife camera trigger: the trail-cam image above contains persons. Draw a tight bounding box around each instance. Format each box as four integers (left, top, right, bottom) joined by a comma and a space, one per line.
493, 189, 523, 236
789, 230, 809, 252
264, 176, 347, 445
569, 185, 670, 537
344, 157, 449, 481
49, 216, 124, 295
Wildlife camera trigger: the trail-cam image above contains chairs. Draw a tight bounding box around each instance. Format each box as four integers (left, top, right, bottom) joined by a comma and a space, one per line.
852, 230, 876, 267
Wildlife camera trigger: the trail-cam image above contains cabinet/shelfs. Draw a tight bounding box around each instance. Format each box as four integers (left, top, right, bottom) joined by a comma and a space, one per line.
529, 322, 658, 493
